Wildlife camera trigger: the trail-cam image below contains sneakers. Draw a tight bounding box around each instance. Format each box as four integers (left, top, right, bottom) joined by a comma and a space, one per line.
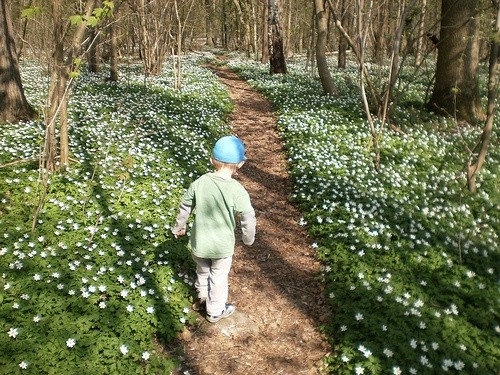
206, 303, 235, 323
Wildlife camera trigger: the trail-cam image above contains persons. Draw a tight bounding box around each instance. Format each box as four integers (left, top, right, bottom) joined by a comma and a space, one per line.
172, 136, 257, 322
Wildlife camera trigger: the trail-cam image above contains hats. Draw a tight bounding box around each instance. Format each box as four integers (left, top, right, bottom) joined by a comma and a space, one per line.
212, 135, 247, 164
